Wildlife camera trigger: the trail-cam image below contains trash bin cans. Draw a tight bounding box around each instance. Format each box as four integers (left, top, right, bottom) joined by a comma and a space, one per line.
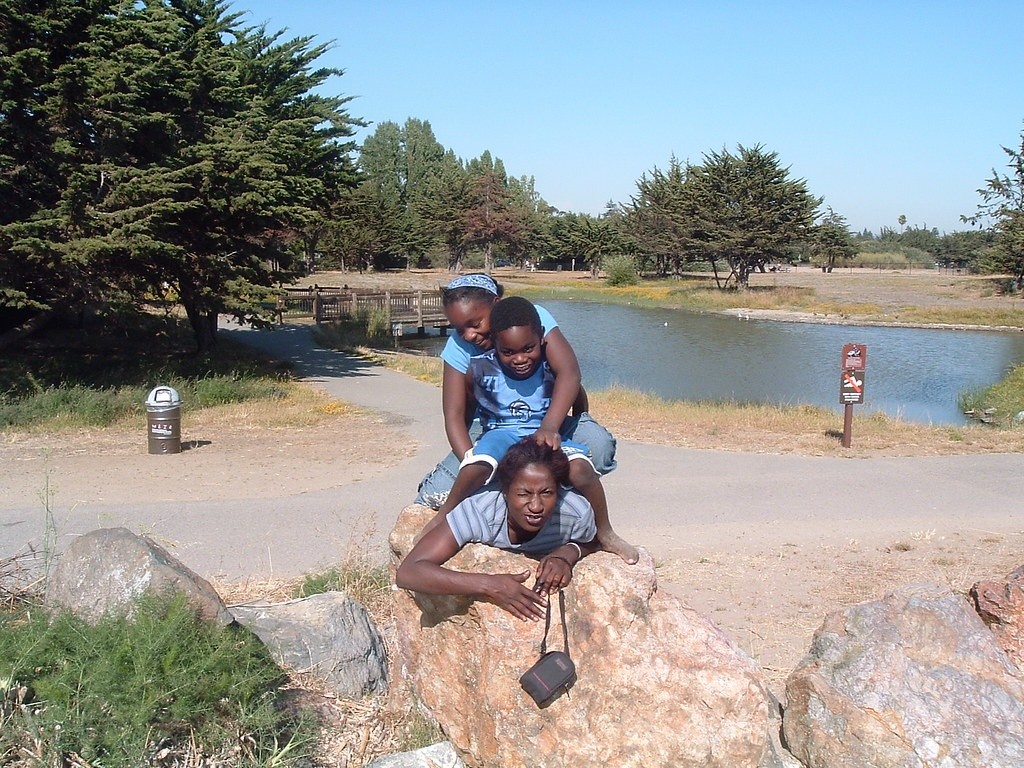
822, 266, 826, 273
145, 386, 182, 454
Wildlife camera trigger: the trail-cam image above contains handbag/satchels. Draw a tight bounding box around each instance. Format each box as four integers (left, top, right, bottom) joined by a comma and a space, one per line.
520, 588, 578, 710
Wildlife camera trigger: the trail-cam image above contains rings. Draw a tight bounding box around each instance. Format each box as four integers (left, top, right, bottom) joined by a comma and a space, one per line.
553, 432, 560, 438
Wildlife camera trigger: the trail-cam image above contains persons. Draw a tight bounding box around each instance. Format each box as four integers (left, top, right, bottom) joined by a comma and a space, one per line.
413, 273, 618, 511
396, 438, 599, 624
413, 296, 639, 565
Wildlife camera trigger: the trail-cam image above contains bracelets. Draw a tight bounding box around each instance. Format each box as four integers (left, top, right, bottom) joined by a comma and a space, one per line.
566, 543, 582, 559
